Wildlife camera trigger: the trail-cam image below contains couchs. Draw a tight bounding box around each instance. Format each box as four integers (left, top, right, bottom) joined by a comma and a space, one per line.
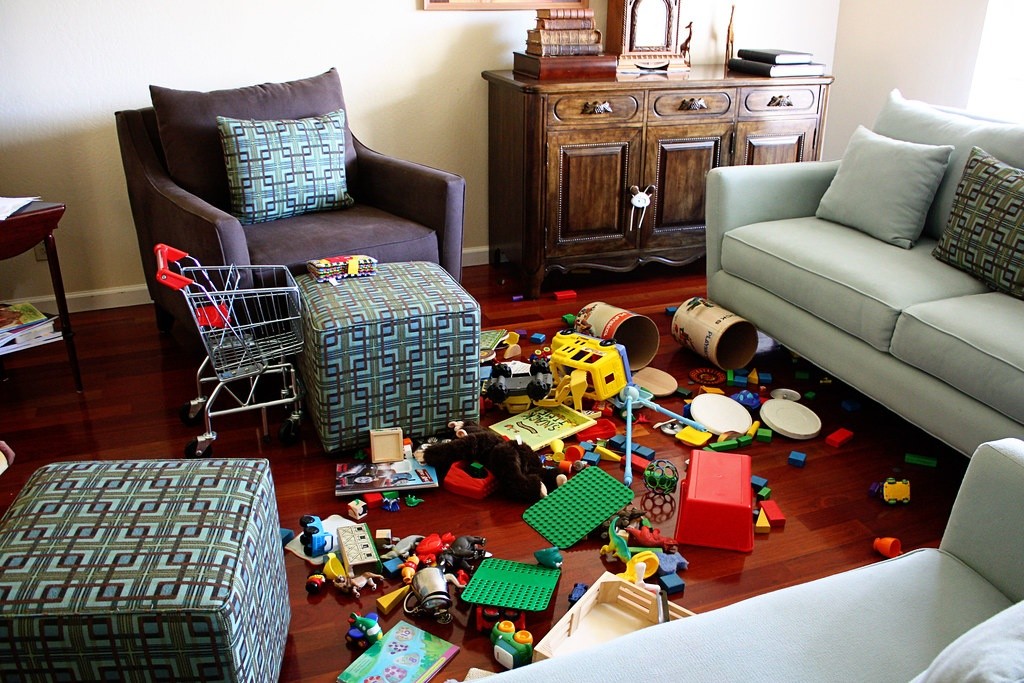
471, 434, 1024, 683
699, 84, 1024, 464
103, 63, 475, 378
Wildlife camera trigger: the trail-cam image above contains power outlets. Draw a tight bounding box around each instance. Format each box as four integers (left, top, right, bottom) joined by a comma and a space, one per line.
33, 241, 47, 262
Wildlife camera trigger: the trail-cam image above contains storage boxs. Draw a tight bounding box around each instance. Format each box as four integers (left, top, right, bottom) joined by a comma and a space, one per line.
528, 570, 703, 662
672, 447, 756, 555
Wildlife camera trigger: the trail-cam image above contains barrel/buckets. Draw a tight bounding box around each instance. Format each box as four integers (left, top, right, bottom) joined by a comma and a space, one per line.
671, 297, 758, 370
573, 302, 660, 371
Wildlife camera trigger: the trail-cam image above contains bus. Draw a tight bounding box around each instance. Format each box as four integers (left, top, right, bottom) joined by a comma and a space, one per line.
547, 329, 636, 401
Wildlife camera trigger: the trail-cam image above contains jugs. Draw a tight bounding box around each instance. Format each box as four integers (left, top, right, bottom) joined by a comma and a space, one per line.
404, 566, 467, 625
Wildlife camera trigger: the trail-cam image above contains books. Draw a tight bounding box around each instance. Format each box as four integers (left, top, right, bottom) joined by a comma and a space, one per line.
488, 402, 597, 453
335, 458, 439, 497
0, 302, 62, 354
727, 50, 826, 77
336, 621, 460, 683
512, 9, 616, 79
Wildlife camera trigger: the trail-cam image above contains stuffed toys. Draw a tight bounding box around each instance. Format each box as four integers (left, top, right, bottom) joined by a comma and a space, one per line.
412, 420, 567, 500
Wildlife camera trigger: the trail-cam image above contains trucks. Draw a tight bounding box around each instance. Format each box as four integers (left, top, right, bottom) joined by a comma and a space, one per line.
301, 514, 334, 556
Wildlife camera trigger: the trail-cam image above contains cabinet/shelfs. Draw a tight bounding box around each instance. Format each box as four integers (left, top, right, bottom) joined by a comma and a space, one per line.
483, 65, 835, 299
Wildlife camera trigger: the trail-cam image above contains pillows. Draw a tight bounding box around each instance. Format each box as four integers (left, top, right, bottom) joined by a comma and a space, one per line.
145, 65, 372, 216
810, 123, 954, 250
209, 106, 356, 226
929, 144, 1024, 301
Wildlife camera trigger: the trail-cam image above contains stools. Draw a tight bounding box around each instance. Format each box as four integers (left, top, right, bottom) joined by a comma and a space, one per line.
294, 255, 486, 456
0, 455, 291, 683
0, 196, 87, 397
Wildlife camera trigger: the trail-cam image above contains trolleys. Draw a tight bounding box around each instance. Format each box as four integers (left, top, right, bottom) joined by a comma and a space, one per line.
153, 244, 308, 458
